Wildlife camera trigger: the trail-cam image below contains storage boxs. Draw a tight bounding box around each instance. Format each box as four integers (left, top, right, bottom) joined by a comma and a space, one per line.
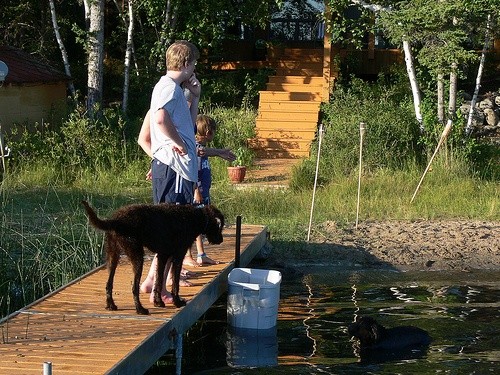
227, 268, 282, 331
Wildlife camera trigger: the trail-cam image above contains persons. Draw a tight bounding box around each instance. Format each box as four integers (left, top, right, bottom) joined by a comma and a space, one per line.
140, 40, 201, 305
183, 114, 238, 267
137, 78, 206, 287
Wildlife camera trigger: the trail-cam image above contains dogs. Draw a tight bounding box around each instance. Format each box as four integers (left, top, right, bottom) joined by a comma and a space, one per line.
76, 197, 226, 317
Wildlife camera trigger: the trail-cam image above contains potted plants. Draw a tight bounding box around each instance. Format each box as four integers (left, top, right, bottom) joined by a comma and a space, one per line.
227, 146, 257, 185
